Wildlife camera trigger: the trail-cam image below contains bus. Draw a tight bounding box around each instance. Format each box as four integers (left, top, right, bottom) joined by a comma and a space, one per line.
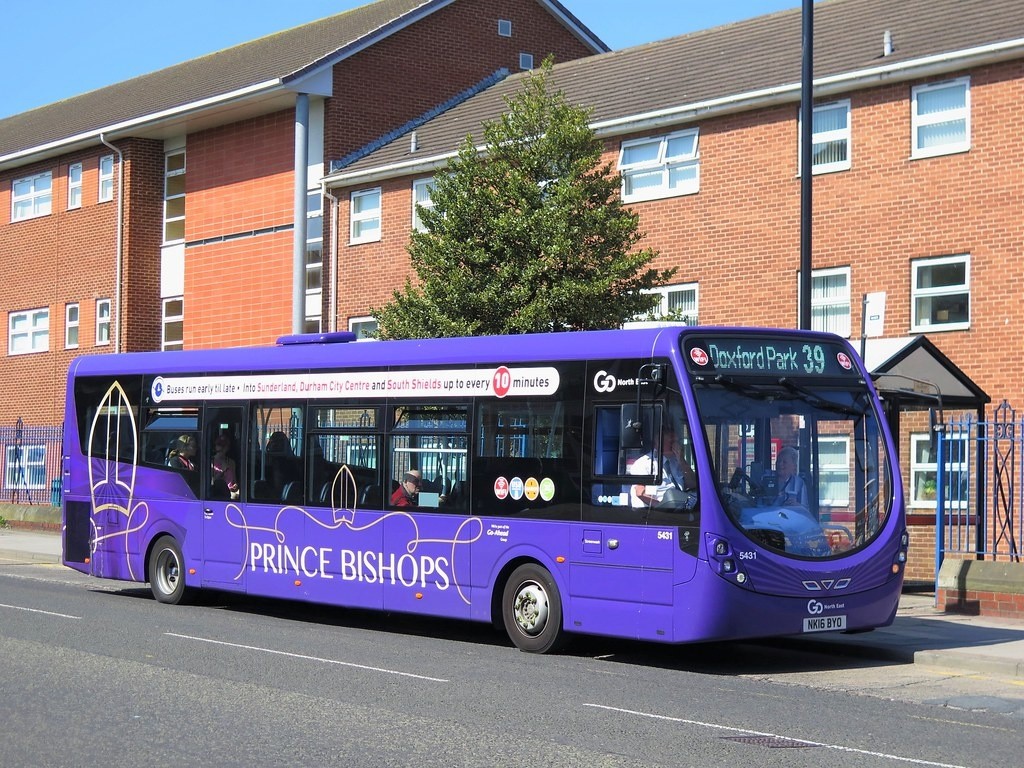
61, 320, 945, 655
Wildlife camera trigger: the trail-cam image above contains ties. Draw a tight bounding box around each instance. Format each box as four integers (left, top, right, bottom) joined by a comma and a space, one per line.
664, 460, 682, 492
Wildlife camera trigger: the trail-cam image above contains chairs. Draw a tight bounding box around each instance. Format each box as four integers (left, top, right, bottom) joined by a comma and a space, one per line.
319, 482, 345, 503
359, 485, 381, 508
164, 440, 178, 467
281, 480, 304, 501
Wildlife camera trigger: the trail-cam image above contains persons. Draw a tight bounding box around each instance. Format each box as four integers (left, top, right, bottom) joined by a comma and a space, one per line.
208, 435, 240, 501
756, 445, 810, 511
390, 468, 448, 508
164, 434, 197, 471
628, 425, 697, 511
